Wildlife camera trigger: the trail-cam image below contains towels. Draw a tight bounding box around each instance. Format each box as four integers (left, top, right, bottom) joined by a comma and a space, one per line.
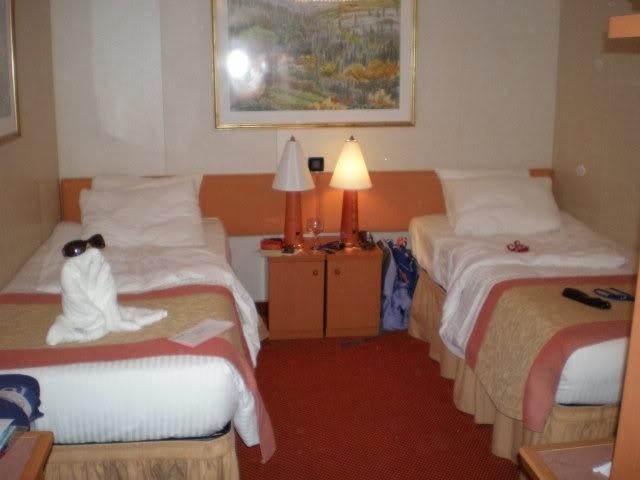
45, 235, 169, 348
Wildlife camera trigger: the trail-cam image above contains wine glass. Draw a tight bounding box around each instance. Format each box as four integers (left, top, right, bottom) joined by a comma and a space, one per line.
307, 218, 323, 251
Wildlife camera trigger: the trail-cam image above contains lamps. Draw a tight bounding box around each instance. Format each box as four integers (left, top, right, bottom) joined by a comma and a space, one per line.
270, 135, 315, 251
329, 134, 372, 248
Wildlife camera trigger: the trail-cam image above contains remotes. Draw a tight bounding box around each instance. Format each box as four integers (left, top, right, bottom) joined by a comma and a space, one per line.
562, 288, 611, 309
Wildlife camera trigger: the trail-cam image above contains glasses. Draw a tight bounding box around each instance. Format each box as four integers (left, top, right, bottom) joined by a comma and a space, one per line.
61, 233, 107, 258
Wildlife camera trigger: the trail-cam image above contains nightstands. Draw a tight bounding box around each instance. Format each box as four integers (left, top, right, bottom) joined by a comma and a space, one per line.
326, 244, 384, 339
267, 247, 325, 341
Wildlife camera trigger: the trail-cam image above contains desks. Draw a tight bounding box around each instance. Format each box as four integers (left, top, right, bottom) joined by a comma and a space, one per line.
516, 435, 616, 480
0, 431, 55, 479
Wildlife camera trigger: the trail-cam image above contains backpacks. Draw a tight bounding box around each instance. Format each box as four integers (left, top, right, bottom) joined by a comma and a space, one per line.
378, 238, 415, 331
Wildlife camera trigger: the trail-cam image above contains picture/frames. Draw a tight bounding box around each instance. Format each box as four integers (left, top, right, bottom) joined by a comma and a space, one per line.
210, 0, 418, 129
0, 0, 22, 145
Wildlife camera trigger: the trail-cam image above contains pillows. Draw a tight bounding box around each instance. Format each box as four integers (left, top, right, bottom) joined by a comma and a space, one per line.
91, 168, 206, 191
77, 179, 208, 247
434, 165, 531, 185
443, 176, 561, 237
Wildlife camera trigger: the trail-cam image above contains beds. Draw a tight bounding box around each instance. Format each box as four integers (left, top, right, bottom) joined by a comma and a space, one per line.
409, 209, 640, 464
0, 218, 277, 479
